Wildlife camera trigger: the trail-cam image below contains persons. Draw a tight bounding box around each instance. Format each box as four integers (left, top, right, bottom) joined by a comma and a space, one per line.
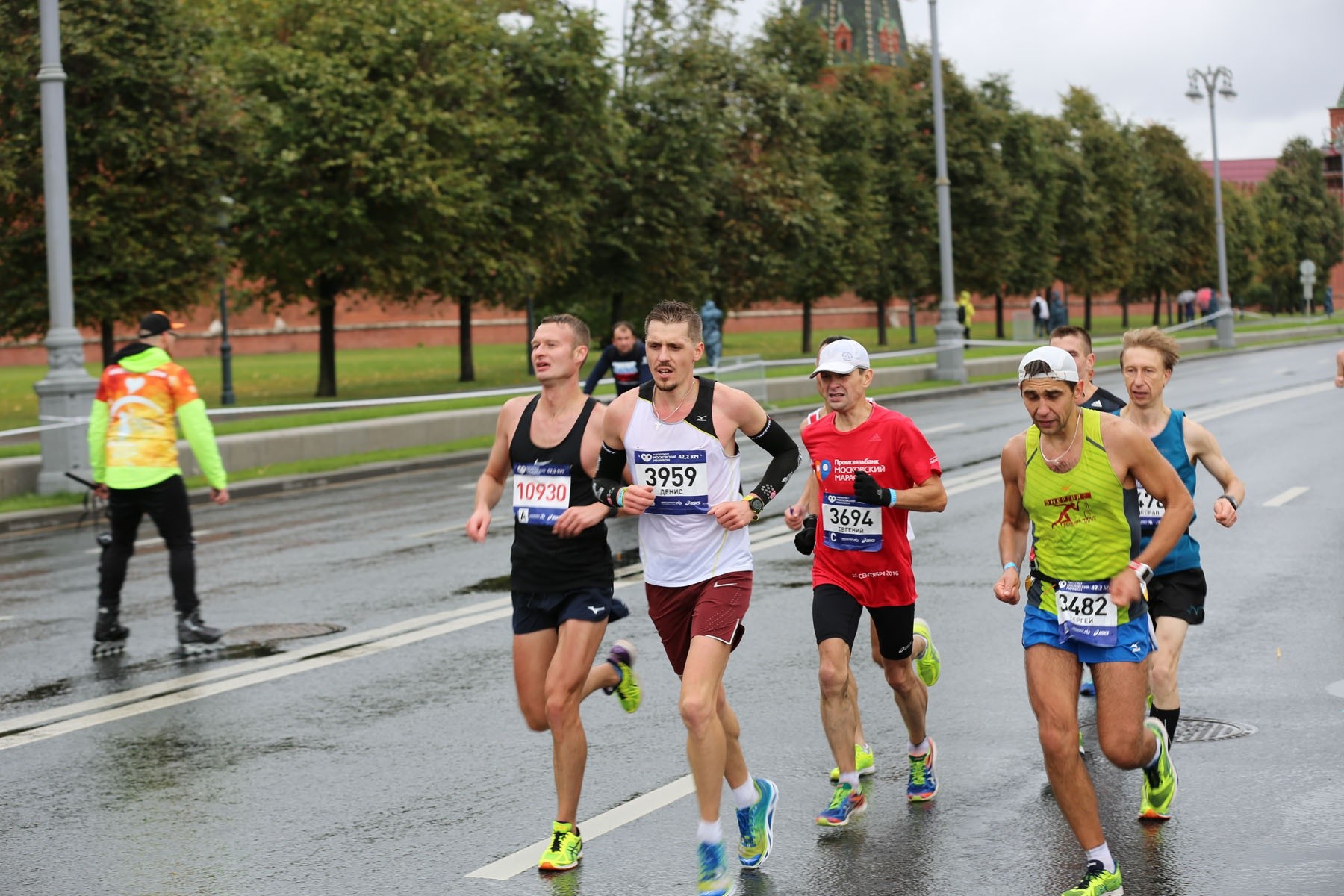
783, 336, 949, 830
88, 314, 230, 660
992, 346, 1194, 896
466, 311, 640, 874
1048, 325, 1154, 757
957, 290, 975, 350
1030, 290, 1050, 339
582, 321, 654, 487
1198, 299, 1210, 319
1323, 286, 1334, 322
1184, 301, 1194, 323
590, 302, 803, 896
1100, 327, 1247, 756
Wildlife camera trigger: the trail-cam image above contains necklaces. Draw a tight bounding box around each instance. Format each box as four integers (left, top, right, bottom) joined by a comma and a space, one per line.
652, 376, 696, 423
1036, 405, 1082, 461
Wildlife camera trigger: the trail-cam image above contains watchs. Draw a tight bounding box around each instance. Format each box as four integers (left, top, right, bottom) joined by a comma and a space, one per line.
742, 496, 764, 514
1217, 494, 1238, 512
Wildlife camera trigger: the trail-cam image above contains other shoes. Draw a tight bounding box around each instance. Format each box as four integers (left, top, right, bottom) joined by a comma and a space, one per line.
1081, 680, 1095, 696
1079, 733, 1085, 755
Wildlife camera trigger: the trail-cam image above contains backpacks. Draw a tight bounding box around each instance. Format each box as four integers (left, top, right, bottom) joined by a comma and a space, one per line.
958, 306, 965, 322
1033, 302, 1040, 315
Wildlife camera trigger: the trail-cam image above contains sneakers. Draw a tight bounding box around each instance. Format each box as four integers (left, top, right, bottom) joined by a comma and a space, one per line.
908, 737, 938, 802
1062, 860, 1124, 896
608, 639, 641, 713
815, 781, 868, 827
1138, 716, 1179, 818
695, 838, 735, 896
735, 778, 779, 868
830, 745, 875, 779
915, 618, 941, 687
538, 820, 584, 870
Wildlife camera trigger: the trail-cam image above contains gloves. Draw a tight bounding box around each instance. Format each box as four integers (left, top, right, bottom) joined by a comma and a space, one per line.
793, 514, 816, 555
854, 470, 898, 508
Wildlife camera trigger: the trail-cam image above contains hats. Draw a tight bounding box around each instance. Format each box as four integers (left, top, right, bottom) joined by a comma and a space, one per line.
1017, 345, 1079, 385
811, 340, 870, 376
138, 311, 186, 339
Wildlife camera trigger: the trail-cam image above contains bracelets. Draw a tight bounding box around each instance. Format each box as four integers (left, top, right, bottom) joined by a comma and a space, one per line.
888, 488, 898, 509
617, 486, 627, 507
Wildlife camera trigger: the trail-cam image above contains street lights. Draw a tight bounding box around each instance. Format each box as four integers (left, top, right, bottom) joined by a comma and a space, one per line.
1183, 62, 1240, 348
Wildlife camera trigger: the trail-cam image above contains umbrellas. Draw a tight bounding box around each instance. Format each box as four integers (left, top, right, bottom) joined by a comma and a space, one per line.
1196, 287, 1211, 302
1176, 289, 1194, 303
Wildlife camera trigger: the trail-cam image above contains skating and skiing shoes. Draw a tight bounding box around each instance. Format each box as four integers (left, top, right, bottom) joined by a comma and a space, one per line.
91, 610, 130, 659
178, 614, 226, 655
